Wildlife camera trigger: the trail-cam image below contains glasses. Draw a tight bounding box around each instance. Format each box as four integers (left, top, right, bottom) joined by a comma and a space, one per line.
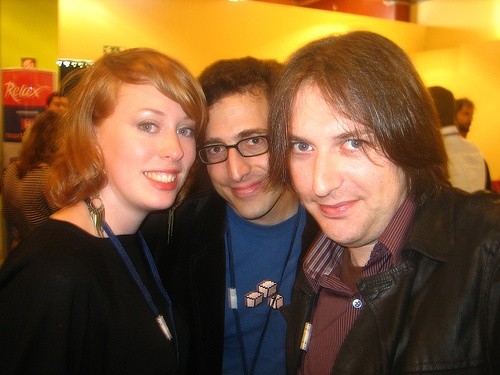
197, 134, 273, 164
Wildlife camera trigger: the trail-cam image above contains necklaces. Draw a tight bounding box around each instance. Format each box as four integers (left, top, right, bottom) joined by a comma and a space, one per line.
84, 195, 181, 375
300, 291, 319, 375
225, 197, 301, 375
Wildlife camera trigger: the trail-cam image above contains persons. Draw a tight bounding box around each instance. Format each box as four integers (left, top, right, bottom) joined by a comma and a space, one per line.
46, 91, 69, 117
2, 110, 62, 256
0, 48, 209, 375
428, 86, 486, 193
454, 99, 492, 191
138, 56, 321, 375
259, 31, 500, 375
